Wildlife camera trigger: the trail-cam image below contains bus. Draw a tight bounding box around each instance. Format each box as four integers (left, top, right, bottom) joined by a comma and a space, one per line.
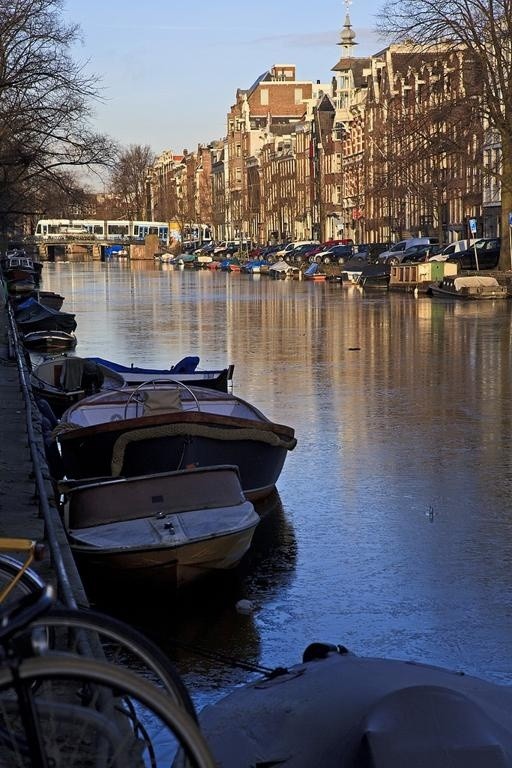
33, 219, 214, 248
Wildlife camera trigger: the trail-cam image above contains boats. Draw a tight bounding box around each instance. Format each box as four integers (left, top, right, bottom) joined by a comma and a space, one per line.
303, 261, 507, 300
154, 252, 302, 279
1, 250, 78, 351
